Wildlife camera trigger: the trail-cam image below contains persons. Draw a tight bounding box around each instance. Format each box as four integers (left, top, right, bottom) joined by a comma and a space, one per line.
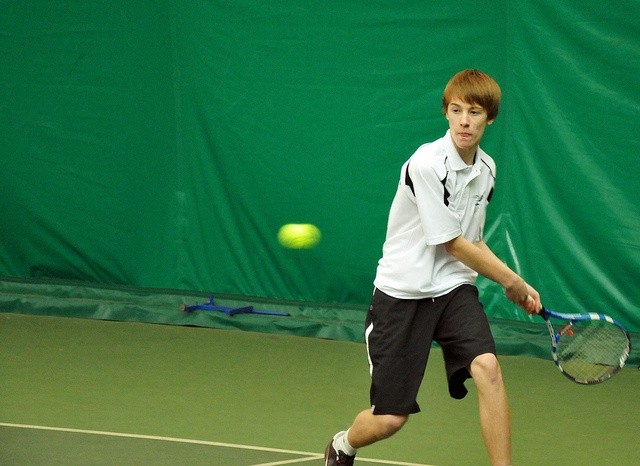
325, 69, 541, 466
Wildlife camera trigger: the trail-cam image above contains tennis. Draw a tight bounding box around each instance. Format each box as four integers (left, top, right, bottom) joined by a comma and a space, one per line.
277, 223, 322, 250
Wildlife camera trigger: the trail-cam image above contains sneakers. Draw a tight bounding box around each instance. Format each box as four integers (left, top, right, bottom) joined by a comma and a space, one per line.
324, 430, 355, 466
